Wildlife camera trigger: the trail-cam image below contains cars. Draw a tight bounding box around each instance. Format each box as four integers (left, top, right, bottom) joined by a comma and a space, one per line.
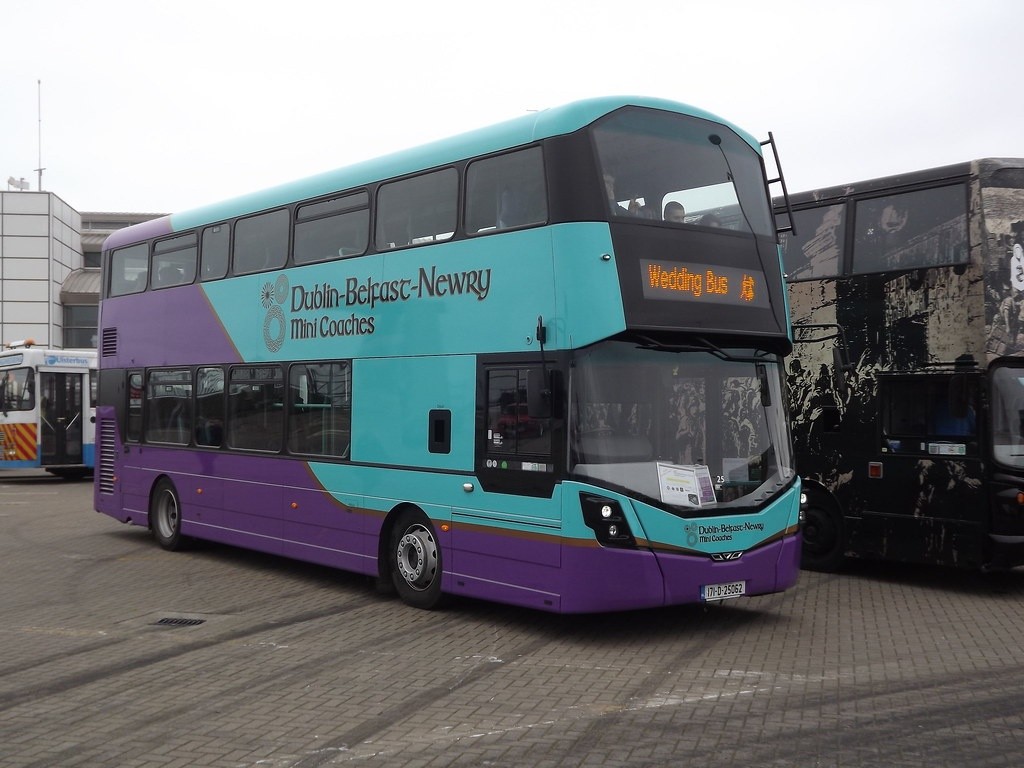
497, 414, 544, 440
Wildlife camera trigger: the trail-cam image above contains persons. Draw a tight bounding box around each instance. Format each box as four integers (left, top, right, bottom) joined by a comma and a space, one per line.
664, 201, 685, 223
628, 201, 641, 217
700, 214, 722, 227
601, 166, 631, 217
637, 206, 653, 219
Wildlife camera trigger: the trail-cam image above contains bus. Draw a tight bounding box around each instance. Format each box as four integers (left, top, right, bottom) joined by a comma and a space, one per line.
575, 159, 1023, 575
92, 98, 857, 612
0, 345, 225, 478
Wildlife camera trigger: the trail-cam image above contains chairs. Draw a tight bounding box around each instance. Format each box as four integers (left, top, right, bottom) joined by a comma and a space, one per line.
137, 193, 529, 289
145, 386, 350, 455
